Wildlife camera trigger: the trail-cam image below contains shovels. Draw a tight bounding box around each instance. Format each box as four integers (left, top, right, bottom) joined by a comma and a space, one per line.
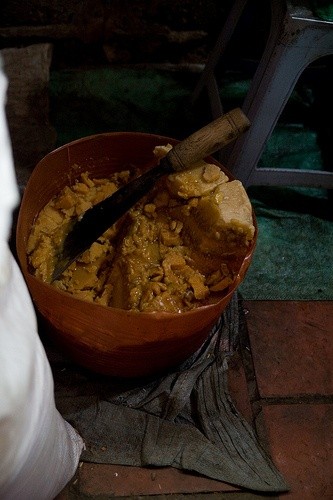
48, 108, 252, 286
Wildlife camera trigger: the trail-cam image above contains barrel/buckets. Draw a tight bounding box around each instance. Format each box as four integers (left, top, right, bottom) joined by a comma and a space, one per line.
15, 131, 256, 379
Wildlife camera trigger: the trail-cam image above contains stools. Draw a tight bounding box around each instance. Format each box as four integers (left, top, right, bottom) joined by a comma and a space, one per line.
198, 0, 333, 227
